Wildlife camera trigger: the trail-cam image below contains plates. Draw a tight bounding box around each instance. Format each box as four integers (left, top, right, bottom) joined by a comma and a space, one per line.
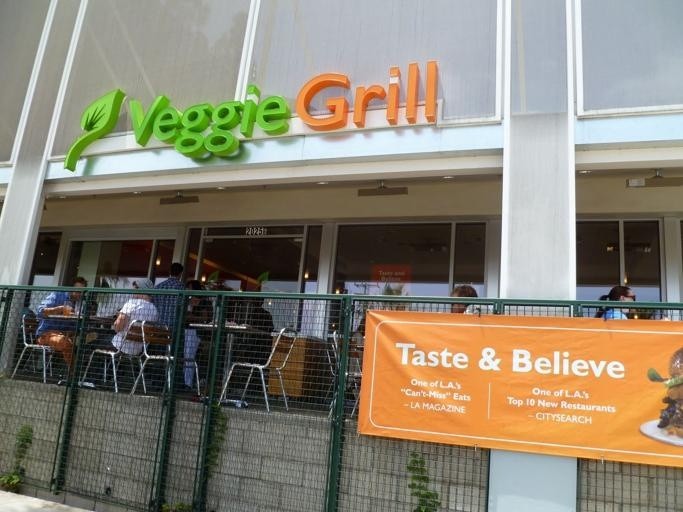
638, 416, 683, 448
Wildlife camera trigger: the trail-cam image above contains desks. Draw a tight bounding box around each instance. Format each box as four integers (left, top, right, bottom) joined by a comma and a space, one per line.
190, 321, 258, 407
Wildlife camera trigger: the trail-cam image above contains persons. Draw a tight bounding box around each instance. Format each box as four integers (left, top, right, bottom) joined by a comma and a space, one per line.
596, 286, 635, 319
77, 280, 158, 389
243, 297, 274, 364
152, 263, 207, 393
450, 285, 492, 314
35, 278, 87, 367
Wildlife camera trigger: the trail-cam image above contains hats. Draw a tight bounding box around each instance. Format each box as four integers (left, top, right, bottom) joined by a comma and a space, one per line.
136, 278, 153, 289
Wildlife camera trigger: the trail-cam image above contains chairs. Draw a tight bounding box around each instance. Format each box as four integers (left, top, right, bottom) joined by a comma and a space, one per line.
9, 311, 172, 401
216, 327, 298, 413
320, 329, 365, 416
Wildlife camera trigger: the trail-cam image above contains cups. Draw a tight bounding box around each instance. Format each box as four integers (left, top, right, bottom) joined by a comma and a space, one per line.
62, 301, 71, 315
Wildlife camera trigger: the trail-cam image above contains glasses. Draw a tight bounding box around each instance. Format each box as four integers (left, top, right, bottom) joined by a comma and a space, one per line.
626, 295, 636, 301
